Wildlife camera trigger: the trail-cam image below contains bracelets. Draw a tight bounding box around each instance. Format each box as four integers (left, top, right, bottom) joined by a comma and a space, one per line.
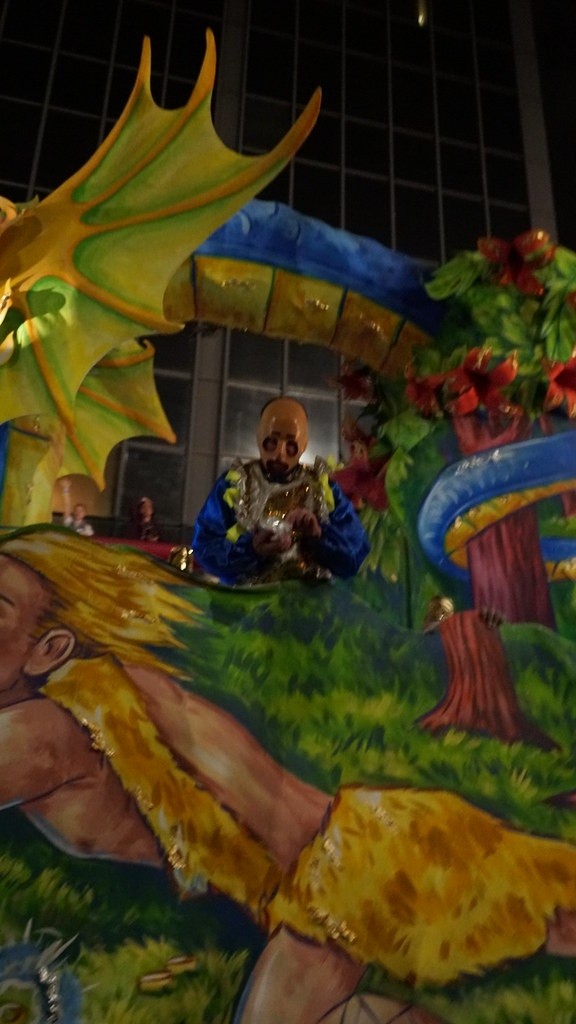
64, 490, 70, 493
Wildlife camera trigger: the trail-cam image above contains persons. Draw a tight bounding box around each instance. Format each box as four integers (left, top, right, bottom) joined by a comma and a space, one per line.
126, 496, 166, 543
190, 395, 370, 588
60, 477, 95, 537
